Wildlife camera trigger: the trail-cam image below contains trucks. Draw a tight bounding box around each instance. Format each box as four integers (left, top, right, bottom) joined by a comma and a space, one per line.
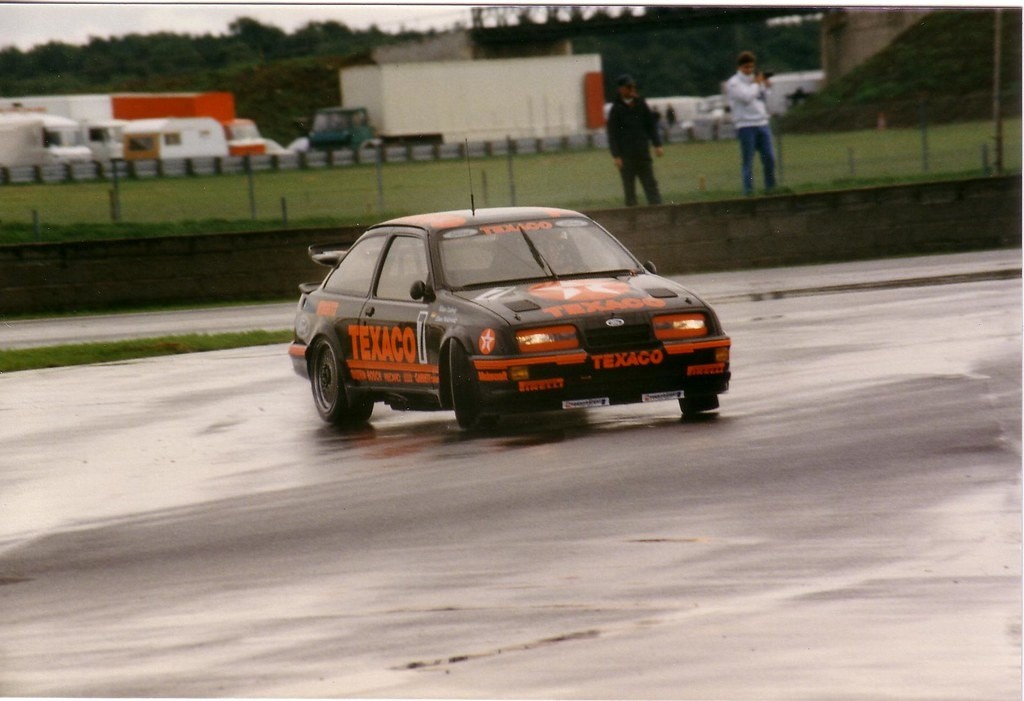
307, 52, 605, 151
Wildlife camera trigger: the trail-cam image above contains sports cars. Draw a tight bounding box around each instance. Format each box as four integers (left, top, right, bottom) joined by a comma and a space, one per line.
288, 205, 733, 434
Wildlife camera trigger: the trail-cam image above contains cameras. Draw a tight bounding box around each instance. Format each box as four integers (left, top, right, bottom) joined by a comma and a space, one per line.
764, 70, 776, 80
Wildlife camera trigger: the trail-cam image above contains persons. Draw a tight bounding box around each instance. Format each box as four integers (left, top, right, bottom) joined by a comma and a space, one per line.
724, 53, 776, 196
605, 74, 665, 207
652, 103, 676, 127
521, 227, 568, 278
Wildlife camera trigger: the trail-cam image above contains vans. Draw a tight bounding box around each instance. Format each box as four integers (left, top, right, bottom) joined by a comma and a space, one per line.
0, 89, 307, 164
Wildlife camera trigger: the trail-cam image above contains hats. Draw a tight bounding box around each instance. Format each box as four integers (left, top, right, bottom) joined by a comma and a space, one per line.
614, 74, 636, 87
